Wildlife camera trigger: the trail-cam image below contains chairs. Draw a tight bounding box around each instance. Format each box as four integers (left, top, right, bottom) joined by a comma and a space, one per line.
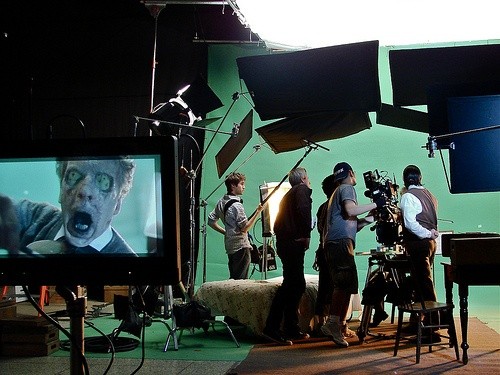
382, 254, 459, 364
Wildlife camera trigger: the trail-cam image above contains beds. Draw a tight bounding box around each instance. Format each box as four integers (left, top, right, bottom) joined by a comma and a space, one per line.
197, 275, 322, 336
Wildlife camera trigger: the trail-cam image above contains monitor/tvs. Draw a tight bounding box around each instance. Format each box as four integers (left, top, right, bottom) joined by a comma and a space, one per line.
0, 134, 181, 288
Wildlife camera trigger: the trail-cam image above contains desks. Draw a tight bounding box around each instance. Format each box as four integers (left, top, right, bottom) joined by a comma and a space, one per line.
356, 251, 426, 344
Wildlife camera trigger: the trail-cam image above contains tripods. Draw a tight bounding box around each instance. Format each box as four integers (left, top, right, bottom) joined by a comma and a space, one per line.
358, 255, 413, 345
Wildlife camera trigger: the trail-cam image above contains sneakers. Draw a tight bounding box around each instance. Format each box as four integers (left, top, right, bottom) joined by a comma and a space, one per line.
339, 325, 356, 337
320, 320, 349, 347
281, 326, 307, 338
261, 327, 293, 345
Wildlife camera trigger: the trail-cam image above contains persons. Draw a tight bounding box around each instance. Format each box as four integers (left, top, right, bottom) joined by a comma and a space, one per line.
262, 167, 313, 346
207, 172, 265, 325
312, 162, 441, 347
0, 159, 137, 254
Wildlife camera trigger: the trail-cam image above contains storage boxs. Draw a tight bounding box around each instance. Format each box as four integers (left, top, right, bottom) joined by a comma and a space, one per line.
104, 286, 130, 302
0, 305, 60, 356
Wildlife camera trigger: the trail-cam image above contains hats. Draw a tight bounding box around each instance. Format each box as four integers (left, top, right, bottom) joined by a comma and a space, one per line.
334, 163, 351, 182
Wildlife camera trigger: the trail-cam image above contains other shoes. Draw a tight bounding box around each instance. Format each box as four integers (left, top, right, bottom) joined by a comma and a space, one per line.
309, 328, 326, 338
370, 310, 388, 328
411, 334, 438, 344
399, 321, 418, 334
